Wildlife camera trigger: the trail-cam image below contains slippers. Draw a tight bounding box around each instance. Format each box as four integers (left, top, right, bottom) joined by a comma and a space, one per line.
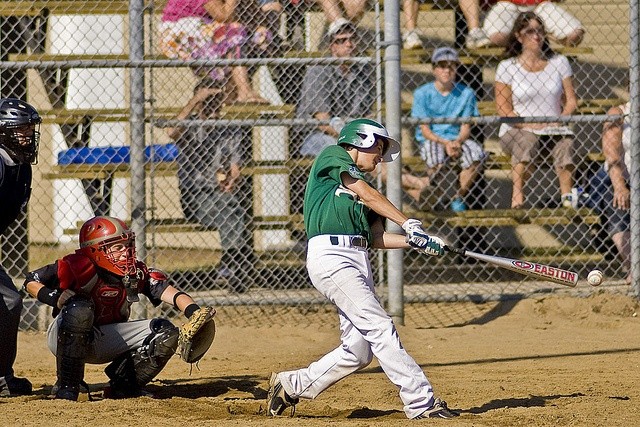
235, 90, 271, 104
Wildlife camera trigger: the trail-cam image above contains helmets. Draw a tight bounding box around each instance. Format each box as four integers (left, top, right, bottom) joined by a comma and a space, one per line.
337, 118, 402, 163
0, 98, 42, 166
79, 215, 138, 277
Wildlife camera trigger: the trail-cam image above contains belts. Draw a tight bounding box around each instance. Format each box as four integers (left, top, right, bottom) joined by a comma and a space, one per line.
330, 236, 369, 247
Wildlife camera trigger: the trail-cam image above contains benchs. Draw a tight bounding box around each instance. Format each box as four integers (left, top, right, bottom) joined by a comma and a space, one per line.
0, 49, 592, 108
44, 151, 631, 199
65, 199, 633, 248
0, 3, 589, 54
33, 90, 625, 150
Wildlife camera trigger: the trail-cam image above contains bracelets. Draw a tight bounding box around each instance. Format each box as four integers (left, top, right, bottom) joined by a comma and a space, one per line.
39, 285, 58, 307
184, 304, 199, 319
174, 291, 191, 309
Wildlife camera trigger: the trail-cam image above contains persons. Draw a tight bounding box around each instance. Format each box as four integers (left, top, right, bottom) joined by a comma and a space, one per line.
482, 0, 586, 48
264, 116, 465, 418
315, 1, 369, 48
22, 214, 217, 399
400, 1, 492, 48
291, 16, 459, 210
408, 46, 485, 211
156, 0, 271, 107
493, 11, 582, 223
164, 76, 257, 295
0, 97, 44, 395
591, 99, 630, 279
255, 0, 301, 39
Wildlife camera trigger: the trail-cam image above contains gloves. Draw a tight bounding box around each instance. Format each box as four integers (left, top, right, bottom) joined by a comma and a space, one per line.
56, 288, 76, 309
402, 219, 429, 250
417, 235, 446, 258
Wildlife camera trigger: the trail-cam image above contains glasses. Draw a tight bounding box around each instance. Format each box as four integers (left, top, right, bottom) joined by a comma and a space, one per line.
525, 27, 544, 34
330, 38, 358, 44
438, 60, 461, 67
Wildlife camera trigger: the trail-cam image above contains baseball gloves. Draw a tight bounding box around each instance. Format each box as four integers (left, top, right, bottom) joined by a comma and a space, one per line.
171, 306, 216, 376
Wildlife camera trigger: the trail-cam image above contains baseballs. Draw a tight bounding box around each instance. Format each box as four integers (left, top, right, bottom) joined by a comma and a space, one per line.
586, 270, 604, 286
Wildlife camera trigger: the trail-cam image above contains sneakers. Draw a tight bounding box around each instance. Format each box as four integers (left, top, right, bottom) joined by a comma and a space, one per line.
414, 397, 460, 420
266, 371, 299, 418
465, 28, 491, 50
0, 371, 32, 398
50, 378, 80, 400
402, 29, 423, 50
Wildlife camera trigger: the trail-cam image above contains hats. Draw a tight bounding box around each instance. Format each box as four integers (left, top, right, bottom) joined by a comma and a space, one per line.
327, 17, 357, 37
431, 46, 459, 66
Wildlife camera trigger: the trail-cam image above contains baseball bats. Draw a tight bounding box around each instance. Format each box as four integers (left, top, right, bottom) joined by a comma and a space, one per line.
405, 233, 579, 287
